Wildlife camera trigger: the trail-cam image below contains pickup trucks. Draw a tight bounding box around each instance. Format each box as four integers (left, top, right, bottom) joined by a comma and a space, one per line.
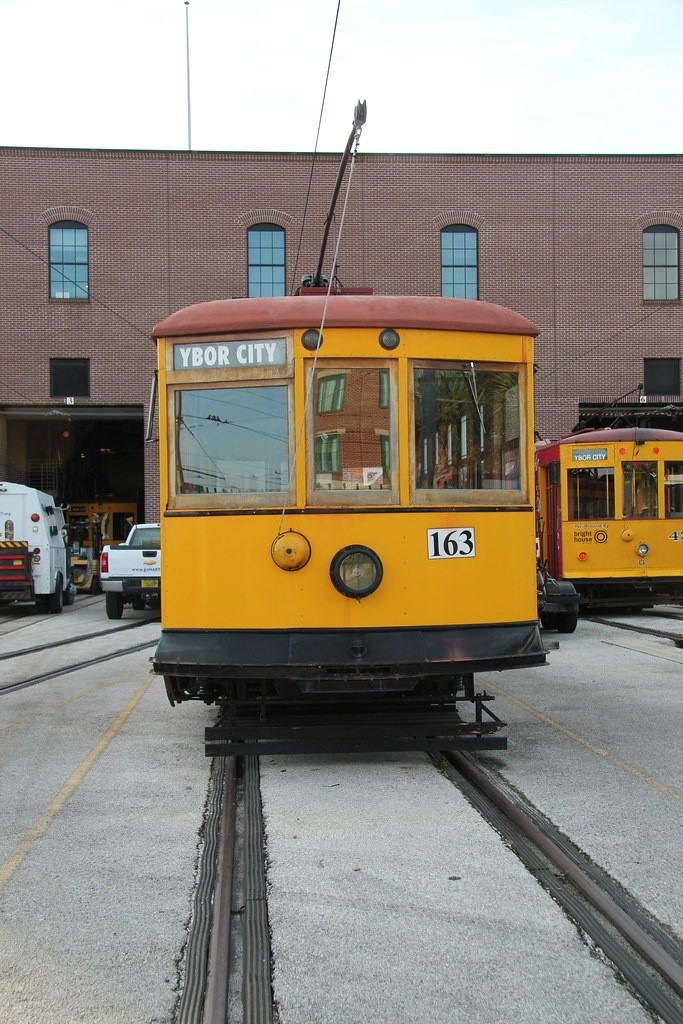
99, 523, 162, 620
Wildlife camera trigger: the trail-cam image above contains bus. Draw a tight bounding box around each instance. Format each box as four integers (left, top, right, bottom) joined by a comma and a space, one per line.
151, 286, 549, 760
505, 427, 683, 616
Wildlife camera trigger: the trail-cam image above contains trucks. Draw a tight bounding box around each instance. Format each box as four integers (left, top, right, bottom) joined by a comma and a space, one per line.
0, 481, 76, 616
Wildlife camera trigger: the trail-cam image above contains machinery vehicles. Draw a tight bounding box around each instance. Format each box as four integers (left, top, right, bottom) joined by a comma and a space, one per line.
69, 513, 103, 594
